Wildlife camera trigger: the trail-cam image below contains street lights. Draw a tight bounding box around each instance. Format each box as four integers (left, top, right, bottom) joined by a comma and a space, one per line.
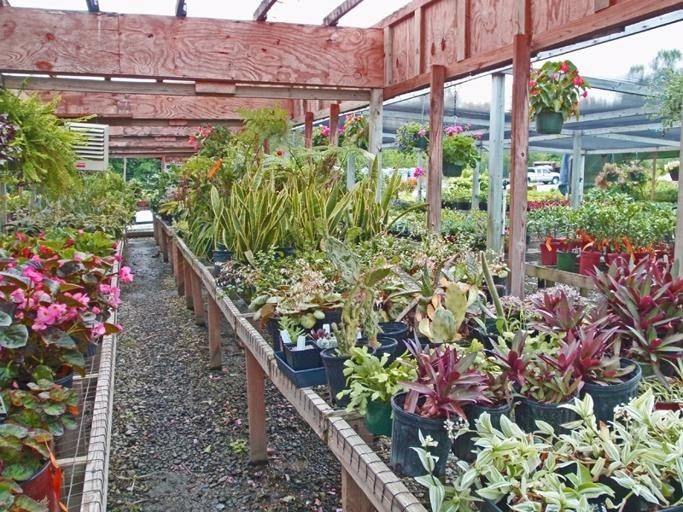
451, 88, 458, 116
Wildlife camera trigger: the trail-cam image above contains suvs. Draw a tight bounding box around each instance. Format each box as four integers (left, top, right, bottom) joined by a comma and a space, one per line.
524, 165, 559, 185
532, 160, 559, 173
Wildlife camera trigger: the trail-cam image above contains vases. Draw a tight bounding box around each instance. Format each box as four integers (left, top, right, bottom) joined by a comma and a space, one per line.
536, 112, 563, 134
20, 372, 74, 391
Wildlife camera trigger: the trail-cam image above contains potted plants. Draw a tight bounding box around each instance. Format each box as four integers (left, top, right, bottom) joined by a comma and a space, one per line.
1, 365, 80, 512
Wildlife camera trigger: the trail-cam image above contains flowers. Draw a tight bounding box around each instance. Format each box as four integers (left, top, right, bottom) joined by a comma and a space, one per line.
0, 250, 135, 379
529, 60, 591, 124
418, 122, 489, 168
